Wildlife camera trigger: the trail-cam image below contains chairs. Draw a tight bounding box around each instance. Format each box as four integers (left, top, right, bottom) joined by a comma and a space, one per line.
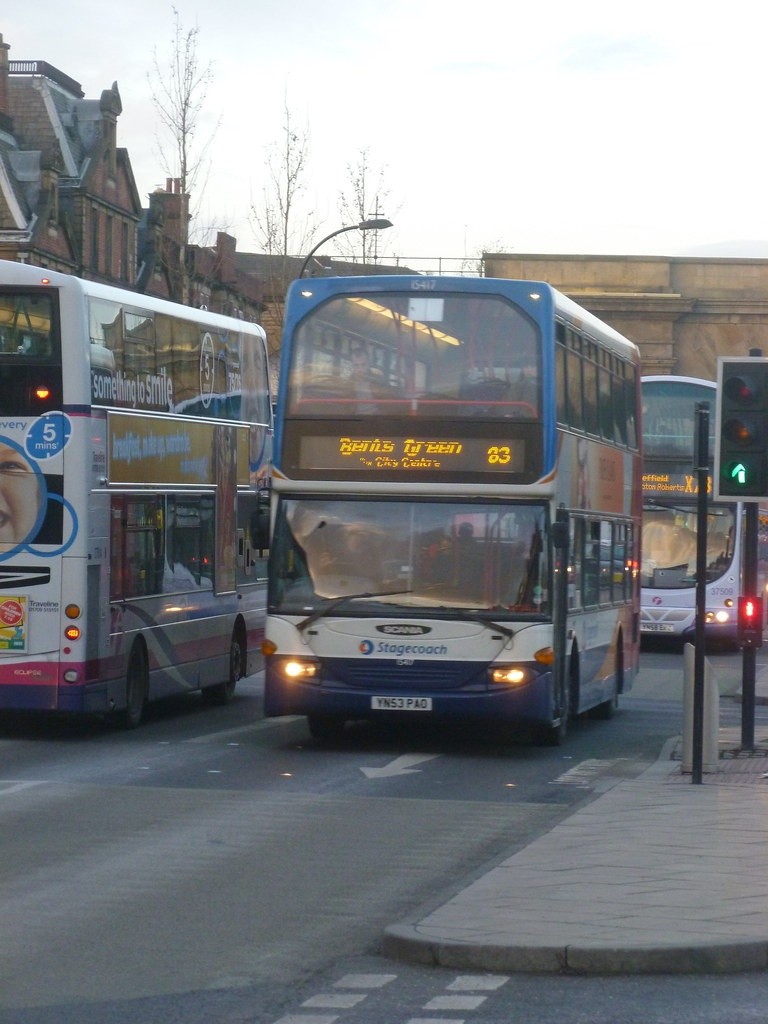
304, 363, 527, 405
647, 413, 686, 448
377, 538, 524, 594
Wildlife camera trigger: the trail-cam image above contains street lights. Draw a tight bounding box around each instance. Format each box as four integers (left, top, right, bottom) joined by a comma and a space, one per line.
299, 219, 394, 279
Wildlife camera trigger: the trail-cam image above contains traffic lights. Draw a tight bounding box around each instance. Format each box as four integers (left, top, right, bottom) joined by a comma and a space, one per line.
713, 357, 768, 503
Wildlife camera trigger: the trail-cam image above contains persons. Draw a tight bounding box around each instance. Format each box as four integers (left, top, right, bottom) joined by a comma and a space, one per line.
348, 349, 395, 416
455, 523, 484, 573
0, 441, 39, 554
320, 529, 381, 576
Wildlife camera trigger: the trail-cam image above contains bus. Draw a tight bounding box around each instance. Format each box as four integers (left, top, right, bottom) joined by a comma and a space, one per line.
249, 275, 645, 749
0, 258, 275, 730
641, 373, 768, 651
